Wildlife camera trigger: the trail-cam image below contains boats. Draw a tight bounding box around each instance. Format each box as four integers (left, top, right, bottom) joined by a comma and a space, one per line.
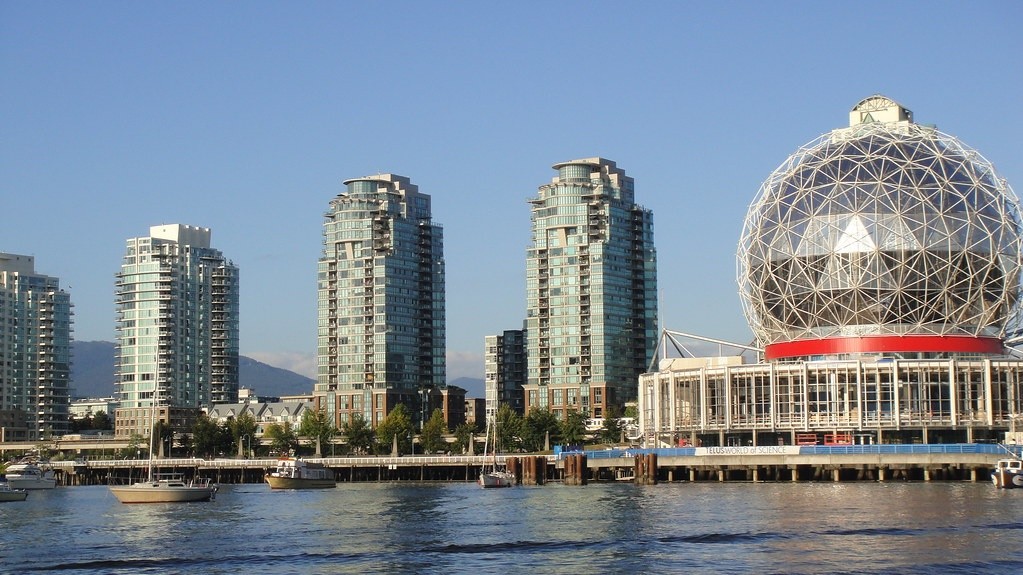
0, 482, 29, 504
5, 455, 57, 489
478, 376, 517, 489
110, 338, 215, 503
263, 458, 336, 489
991, 439, 1023, 489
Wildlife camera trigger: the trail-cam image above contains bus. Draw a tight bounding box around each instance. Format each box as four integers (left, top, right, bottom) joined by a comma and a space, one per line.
582, 417, 635, 431
